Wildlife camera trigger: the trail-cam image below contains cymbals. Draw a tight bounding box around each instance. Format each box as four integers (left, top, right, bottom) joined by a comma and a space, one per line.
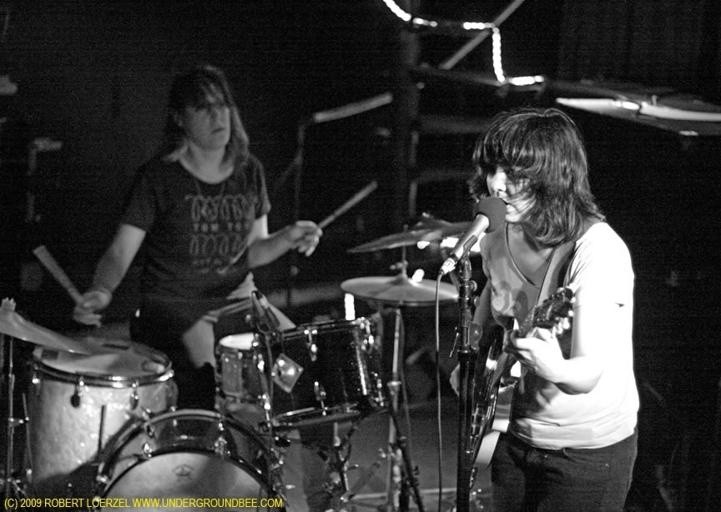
0, 306, 91, 355
340, 276, 464, 308
348, 220, 473, 255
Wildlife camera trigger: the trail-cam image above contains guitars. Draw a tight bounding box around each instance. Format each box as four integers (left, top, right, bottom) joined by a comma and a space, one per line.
466, 284, 577, 470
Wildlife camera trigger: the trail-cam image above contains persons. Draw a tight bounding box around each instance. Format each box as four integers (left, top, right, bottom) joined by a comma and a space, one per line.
422, 105, 645, 511
66, 56, 347, 512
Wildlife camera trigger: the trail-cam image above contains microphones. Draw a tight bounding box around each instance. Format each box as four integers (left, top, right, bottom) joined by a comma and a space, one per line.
255, 291, 282, 348
437, 195, 507, 277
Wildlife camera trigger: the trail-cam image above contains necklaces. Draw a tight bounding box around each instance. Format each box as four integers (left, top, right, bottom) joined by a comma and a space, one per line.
189, 169, 227, 223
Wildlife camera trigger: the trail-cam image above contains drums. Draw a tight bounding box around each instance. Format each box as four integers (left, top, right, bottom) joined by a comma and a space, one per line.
212, 329, 278, 406
258, 318, 389, 433
20, 334, 180, 510
83, 410, 289, 511
